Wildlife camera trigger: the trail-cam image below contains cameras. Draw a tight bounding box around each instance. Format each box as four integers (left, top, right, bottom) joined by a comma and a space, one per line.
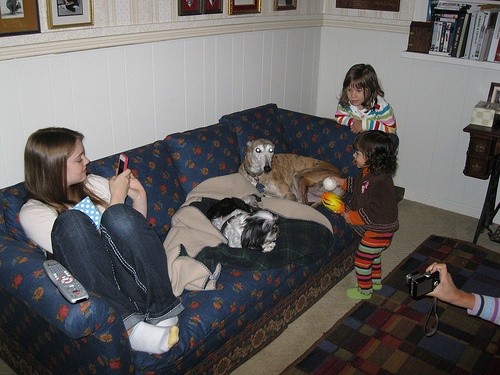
406, 271, 439, 298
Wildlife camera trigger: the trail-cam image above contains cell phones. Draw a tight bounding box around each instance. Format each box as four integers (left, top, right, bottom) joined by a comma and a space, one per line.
117, 153, 128, 175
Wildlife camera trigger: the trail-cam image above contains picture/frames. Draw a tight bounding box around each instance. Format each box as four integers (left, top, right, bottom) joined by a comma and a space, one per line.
178, 0, 223, 17
487, 81, 500, 103
0, 0, 43, 37
45, 0, 95, 30
228, 0, 262, 16
272, 0, 297, 11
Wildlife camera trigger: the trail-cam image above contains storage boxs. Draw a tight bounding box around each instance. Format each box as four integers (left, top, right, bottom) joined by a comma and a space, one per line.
470, 100, 496, 127
407, 21, 434, 54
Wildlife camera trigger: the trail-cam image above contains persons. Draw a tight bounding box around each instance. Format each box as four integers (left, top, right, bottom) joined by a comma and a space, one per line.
19, 127, 185, 354
426, 262, 500, 326
335, 64, 396, 134
331, 130, 400, 300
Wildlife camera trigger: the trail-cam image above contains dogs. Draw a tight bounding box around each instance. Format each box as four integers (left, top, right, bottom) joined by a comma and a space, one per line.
208, 196, 279, 252
238, 139, 347, 203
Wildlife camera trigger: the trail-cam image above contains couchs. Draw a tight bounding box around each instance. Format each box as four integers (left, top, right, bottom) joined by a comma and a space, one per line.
0, 102, 396, 375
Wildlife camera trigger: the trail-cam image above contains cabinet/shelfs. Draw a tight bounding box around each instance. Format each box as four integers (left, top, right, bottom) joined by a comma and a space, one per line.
461, 123, 500, 245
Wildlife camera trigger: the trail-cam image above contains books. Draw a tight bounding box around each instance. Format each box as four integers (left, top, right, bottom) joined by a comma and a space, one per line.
475, 101, 500, 114
406, 0, 500, 63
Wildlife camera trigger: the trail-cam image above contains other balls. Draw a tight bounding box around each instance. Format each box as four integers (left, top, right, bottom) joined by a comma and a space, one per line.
322, 176, 338, 192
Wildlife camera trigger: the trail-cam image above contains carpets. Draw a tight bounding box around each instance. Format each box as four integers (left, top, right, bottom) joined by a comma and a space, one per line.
280, 235, 500, 375
